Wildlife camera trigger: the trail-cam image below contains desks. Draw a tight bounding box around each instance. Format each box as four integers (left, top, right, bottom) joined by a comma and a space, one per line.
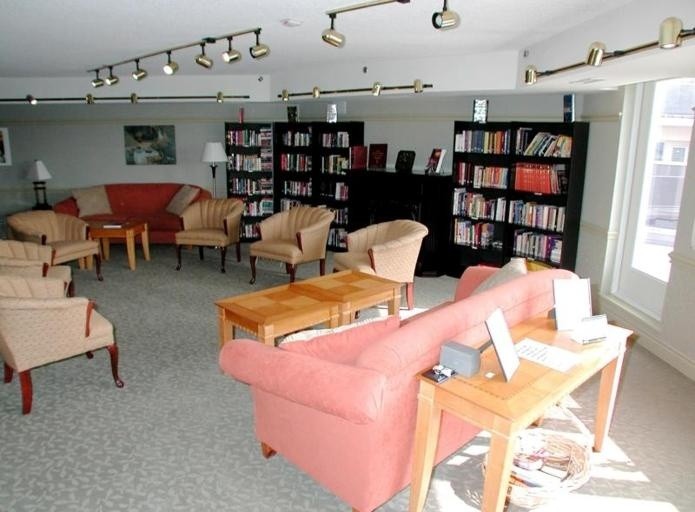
409, 306, 633, 512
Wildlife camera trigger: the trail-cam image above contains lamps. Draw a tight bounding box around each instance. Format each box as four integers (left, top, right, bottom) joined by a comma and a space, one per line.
26, 160, 53, 211
200, 142, 229, 198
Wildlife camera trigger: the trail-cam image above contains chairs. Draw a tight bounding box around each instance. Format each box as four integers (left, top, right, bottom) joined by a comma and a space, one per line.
250, 204, 335, 284
174, 199, 244, 273
0, 210, 124, 415
333, 219, 428, 317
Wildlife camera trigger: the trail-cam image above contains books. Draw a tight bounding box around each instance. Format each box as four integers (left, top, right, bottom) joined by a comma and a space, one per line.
226, 122, 354, 249
495, 447, 575, 491
103, 222, 124, 229
449, 126, 573, 267
428, 148, 447, 173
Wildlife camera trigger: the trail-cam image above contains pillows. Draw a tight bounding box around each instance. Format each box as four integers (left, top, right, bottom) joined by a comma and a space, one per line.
165, 185, 200, 215
468, 257, 527, 295
278, 315, 401, 364
70, 184, 113, 218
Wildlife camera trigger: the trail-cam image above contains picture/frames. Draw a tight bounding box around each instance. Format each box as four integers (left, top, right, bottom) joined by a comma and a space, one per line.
552, 278, 592, 329
485, 307, 519, 382
0, 128, 12, 166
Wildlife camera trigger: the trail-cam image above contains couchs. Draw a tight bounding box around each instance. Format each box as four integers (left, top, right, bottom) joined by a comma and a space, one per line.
51, 182, 211, 251
219, 266, 579, 512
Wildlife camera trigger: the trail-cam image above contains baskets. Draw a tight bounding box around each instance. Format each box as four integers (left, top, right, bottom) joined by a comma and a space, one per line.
482, 427, 590, 508
